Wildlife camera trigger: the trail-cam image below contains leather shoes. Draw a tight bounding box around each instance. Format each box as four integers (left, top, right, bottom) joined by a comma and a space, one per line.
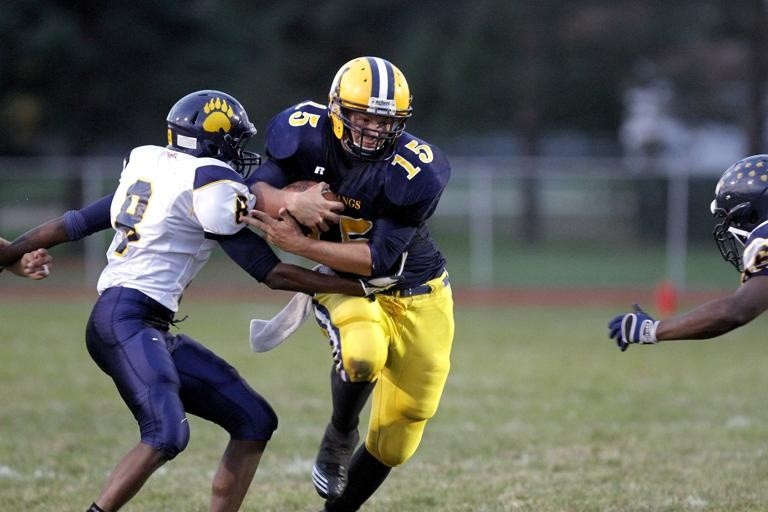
608, 303, 661, 352
358, 274, 406, 298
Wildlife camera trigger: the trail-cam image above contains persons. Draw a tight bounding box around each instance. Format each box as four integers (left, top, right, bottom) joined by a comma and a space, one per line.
0, 237, 55, 282
606, 154, 767, 353
237, 56, 456, 512
1, 88, 434, 511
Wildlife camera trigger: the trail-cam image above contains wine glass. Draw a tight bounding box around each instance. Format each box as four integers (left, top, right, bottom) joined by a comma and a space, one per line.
43, 264, 51, 278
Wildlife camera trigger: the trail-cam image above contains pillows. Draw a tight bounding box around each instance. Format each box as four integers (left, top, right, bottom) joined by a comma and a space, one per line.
710, 153, 768, 274
166, 89, 261, 178
327, 55, 413, 155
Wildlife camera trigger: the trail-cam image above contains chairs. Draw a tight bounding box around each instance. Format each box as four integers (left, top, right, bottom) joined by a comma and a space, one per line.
311, 422, 359, 500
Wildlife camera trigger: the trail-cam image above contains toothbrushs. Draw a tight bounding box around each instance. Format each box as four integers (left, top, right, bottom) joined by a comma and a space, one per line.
280, 180, 338, 233
